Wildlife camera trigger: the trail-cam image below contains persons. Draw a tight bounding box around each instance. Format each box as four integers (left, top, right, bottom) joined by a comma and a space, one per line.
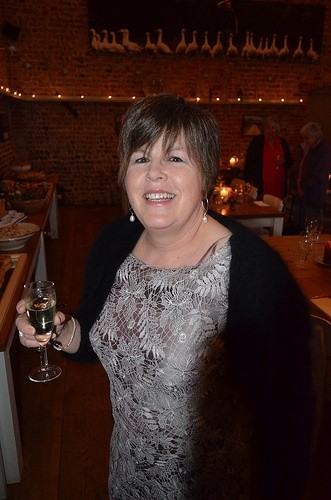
15, 93, 315, 500
296, 121, 331, 236
244, 120, 293, 212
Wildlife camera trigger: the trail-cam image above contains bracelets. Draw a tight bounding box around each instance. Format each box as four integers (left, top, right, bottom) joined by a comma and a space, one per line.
52, 313, 76, 351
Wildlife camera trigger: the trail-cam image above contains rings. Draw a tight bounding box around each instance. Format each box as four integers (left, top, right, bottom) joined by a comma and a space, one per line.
18, 331, 25, 337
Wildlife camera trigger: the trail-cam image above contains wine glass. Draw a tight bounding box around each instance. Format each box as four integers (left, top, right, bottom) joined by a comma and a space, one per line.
24, 280, 62, 382
306, 218, 322, 250
298, 234, 317, 267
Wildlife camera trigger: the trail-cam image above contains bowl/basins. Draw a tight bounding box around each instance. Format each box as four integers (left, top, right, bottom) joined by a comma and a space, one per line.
0, 223, 40, 251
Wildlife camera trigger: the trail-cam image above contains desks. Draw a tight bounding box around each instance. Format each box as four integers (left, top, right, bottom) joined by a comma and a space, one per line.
208, 184, 285, 235
0, 171, 61, 500
262, 234, 331, 324
